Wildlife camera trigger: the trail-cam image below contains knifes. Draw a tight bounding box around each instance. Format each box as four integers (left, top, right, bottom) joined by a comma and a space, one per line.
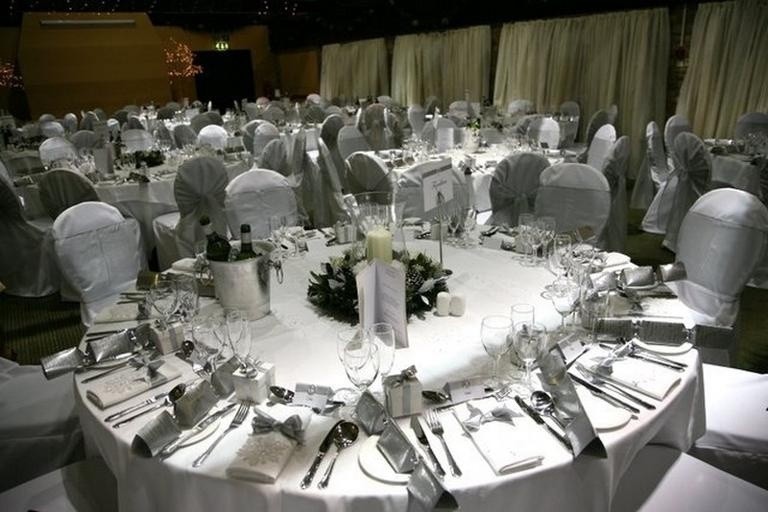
159, 402, 237, 462
599, 336, 685, 372
104, 391, 171, 422
567, 371, 641, 414
514, 396, 575, 455
410, 412, 446, 476
300, 420, 344, 489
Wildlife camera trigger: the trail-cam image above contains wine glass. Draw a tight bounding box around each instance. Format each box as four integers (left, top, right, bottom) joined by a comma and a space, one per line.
513, 213, 610, 346
334, 323, 398, 418
444, 194, 477, 248
147, 273, 200, 329
479, 303, 547, 399
193, 307, 253, 374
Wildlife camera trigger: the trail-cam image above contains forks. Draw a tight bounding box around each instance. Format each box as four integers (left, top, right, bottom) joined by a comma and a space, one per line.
617, 338, 687, 368
577, 363, 656, 411
425, 407, 463, 477
81, 346, 153, 384
193, 397, 252, 467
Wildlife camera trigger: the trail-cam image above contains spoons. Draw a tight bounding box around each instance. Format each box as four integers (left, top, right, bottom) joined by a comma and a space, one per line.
530, 391, 567, 431
180, 340, 211, 373
422, 390, 452, 403
113, 383, 187, 427
318, 421, 360, 489
270, 384, 343, 408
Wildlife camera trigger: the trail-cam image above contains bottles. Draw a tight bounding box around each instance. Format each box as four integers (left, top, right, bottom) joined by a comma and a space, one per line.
199, 215, 232, 262
236, 224, 258, 262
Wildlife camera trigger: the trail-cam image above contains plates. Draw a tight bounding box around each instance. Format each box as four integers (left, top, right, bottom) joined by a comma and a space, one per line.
625, 281, 662, 291
179, 405, 222, 449
575, 386, 632, 430
354, 433, 426, 486
630, 337, 694, 355
